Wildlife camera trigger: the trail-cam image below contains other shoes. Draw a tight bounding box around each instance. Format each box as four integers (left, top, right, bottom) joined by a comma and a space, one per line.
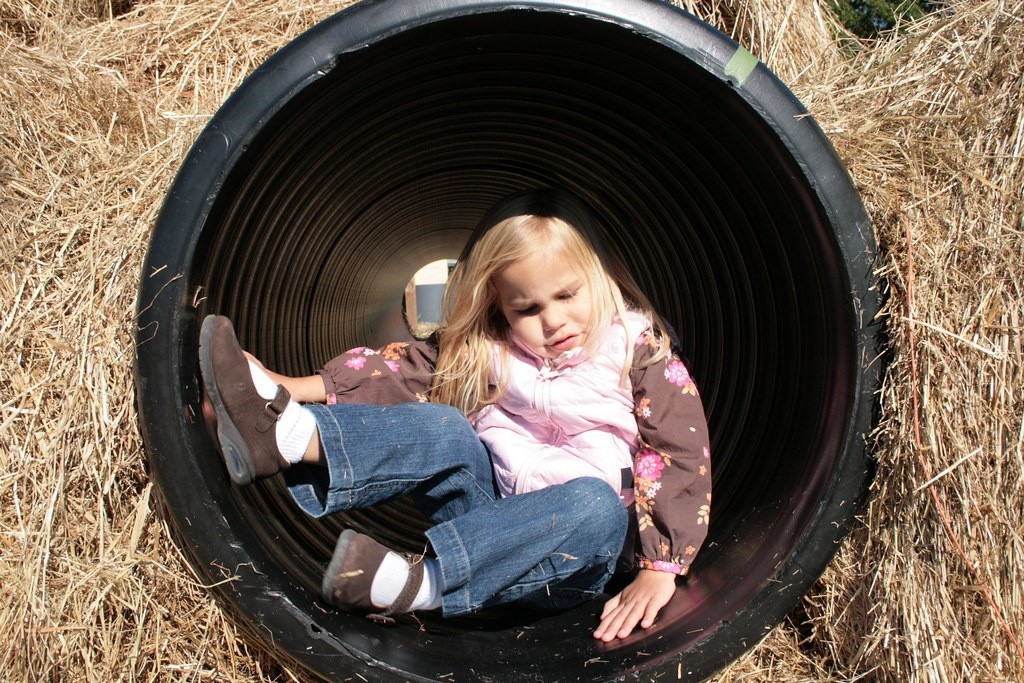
199, 314, 291, 488
321, 531, 425, 626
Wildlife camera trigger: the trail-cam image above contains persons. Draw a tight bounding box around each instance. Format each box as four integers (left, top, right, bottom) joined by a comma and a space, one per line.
196, 185, 711, 639
